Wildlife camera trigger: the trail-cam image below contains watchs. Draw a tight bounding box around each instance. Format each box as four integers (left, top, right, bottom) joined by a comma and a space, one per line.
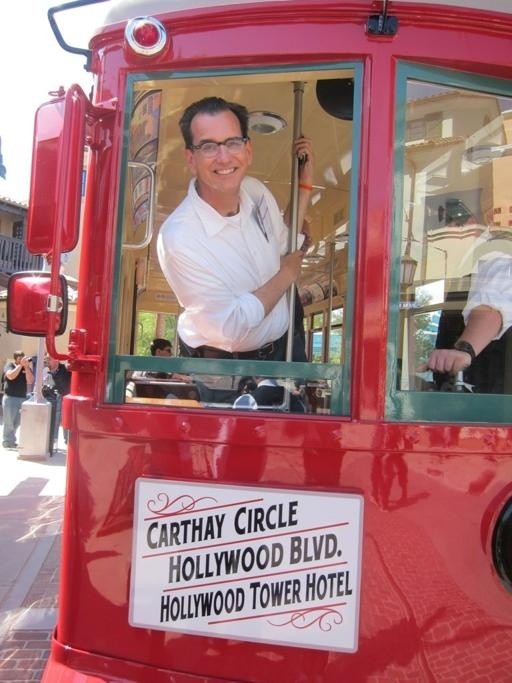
451, 339, 477, 363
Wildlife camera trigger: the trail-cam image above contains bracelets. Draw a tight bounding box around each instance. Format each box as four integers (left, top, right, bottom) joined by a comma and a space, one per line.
298, 182, 313, 191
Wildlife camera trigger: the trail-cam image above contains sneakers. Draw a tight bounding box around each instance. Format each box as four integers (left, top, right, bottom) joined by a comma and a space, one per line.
3, 441, 18, 448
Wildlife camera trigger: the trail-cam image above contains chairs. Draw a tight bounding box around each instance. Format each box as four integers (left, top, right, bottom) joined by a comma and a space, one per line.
126, 375, 200, 400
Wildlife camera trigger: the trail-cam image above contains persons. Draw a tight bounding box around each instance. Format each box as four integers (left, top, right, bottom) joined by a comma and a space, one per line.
1, 355, 73, 449
154, 96, 315, 402
122, 338, 194, 384
234, 374, 309, 415
416, 195, 512, 395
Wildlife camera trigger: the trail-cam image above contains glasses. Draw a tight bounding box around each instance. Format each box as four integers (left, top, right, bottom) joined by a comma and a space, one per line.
189, 137, 247, 158
159, 346, 171, 353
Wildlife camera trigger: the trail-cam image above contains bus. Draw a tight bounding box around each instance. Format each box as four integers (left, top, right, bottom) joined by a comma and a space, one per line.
5, 0, 512, 682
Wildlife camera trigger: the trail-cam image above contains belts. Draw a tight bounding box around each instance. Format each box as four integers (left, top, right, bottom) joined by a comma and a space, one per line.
179, 334, 287, 360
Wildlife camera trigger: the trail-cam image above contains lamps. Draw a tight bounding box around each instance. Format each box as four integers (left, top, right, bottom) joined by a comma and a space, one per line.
244, 111, 286, 136
